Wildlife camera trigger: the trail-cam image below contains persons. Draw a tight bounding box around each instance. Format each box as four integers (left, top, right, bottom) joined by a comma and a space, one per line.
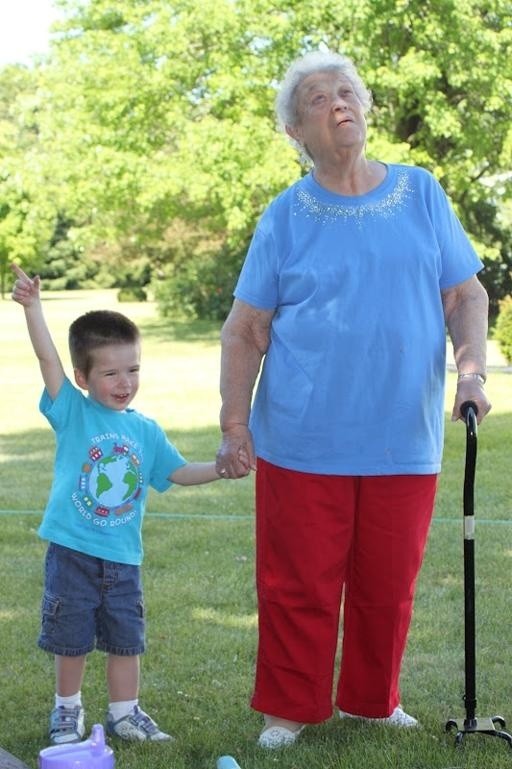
215, 49, 494, 754
10, 260, 253, 748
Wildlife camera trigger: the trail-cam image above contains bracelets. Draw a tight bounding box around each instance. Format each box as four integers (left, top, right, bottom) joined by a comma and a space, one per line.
455, 371, 486, 387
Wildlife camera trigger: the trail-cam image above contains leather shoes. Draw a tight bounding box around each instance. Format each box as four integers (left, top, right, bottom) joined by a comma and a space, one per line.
259, 719, 308, 750
339, 706, 419, 730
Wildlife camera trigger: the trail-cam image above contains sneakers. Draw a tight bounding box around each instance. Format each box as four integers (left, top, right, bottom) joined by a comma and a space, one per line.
49, 703, 86, 745
107, 704, 175, 744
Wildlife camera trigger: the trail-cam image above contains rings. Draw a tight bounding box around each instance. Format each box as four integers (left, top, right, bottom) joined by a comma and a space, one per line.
219, 467, 227, 475
